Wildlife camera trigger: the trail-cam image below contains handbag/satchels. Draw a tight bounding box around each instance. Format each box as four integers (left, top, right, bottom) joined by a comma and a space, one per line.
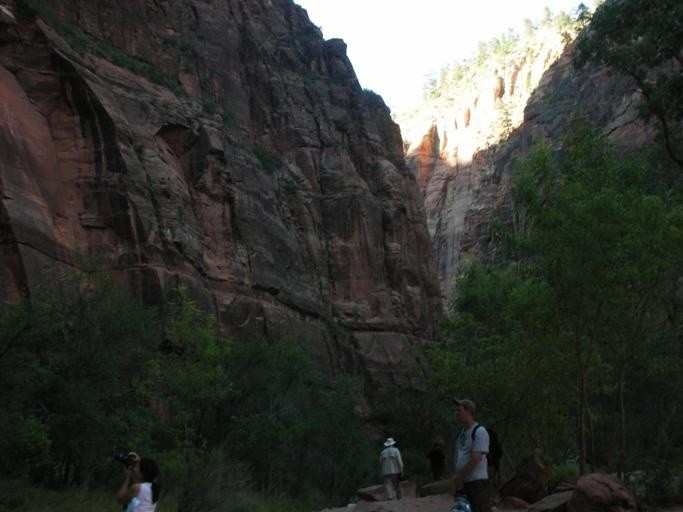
447, 488, 474, 512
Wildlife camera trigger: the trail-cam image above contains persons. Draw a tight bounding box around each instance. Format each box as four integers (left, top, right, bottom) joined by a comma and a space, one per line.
116, 450, 163, 512
426, 441, 446, 481
450, 396, 492, 511
486, 432, 505, 506
378, 436, 406, 501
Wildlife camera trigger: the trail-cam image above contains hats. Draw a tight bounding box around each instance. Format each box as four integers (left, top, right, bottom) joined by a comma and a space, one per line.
452, 396, 476, 414
383, 437, 396, 447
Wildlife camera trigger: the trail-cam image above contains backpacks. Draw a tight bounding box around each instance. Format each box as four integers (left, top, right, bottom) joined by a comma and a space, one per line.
470, 424, 505, 478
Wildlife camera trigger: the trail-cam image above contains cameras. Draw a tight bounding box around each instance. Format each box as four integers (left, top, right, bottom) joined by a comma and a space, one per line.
115, 453, 137, 465
